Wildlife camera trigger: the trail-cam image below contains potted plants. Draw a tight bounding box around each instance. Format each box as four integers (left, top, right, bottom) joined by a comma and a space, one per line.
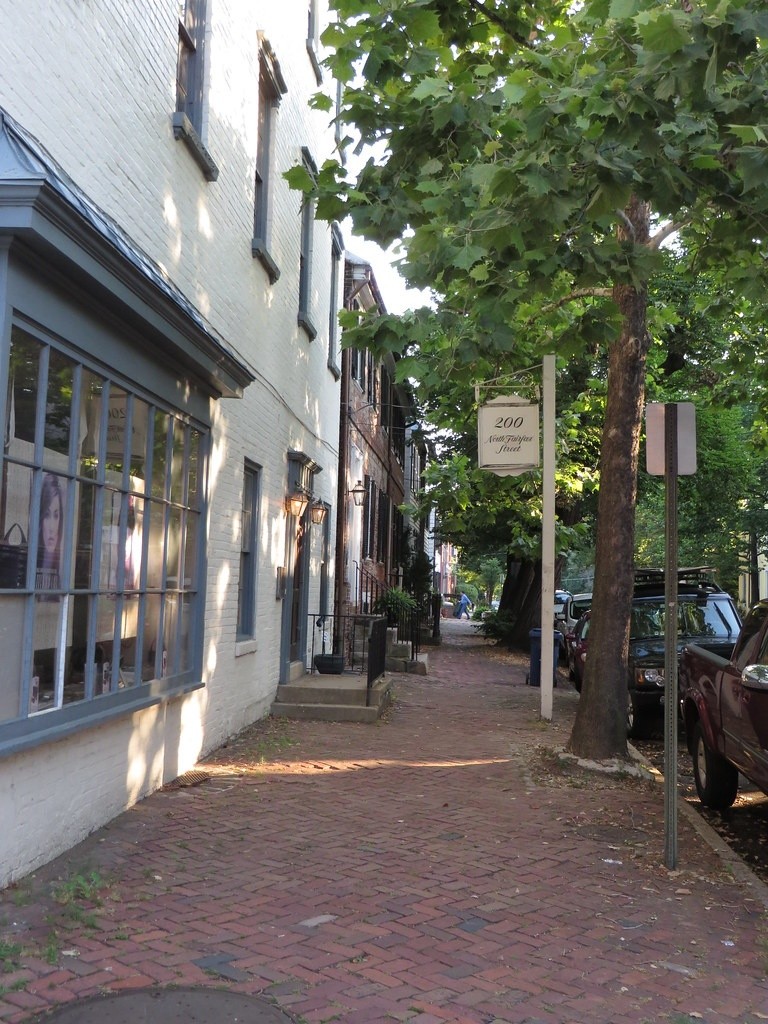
314, 652, 347, 674
371, 586, 418, 626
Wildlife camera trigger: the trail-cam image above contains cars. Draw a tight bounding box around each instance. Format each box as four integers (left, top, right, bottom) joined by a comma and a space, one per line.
564, 608, 592, 692
557, 591, 593, 658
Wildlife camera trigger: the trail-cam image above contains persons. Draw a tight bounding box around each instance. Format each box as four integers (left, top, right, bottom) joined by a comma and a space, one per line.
457, 591, 471, 620
472, 602, 475, 612
109, 493, 138, 598
27, 472, 63, 601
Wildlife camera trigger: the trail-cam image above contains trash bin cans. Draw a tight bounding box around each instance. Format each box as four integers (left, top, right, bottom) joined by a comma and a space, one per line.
525, 630, 564, 687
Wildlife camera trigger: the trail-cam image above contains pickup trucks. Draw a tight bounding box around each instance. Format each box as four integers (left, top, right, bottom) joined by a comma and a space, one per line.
679, 597, 768, 812
628, 592, 743, 741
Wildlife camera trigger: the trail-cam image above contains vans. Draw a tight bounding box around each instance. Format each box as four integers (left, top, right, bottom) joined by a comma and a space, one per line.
552, 590, 574, 628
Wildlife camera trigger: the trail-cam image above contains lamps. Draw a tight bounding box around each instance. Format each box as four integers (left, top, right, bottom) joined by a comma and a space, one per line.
311, 498, 328, 523
284, 488, 309, 517
349, 481, 367, 506
